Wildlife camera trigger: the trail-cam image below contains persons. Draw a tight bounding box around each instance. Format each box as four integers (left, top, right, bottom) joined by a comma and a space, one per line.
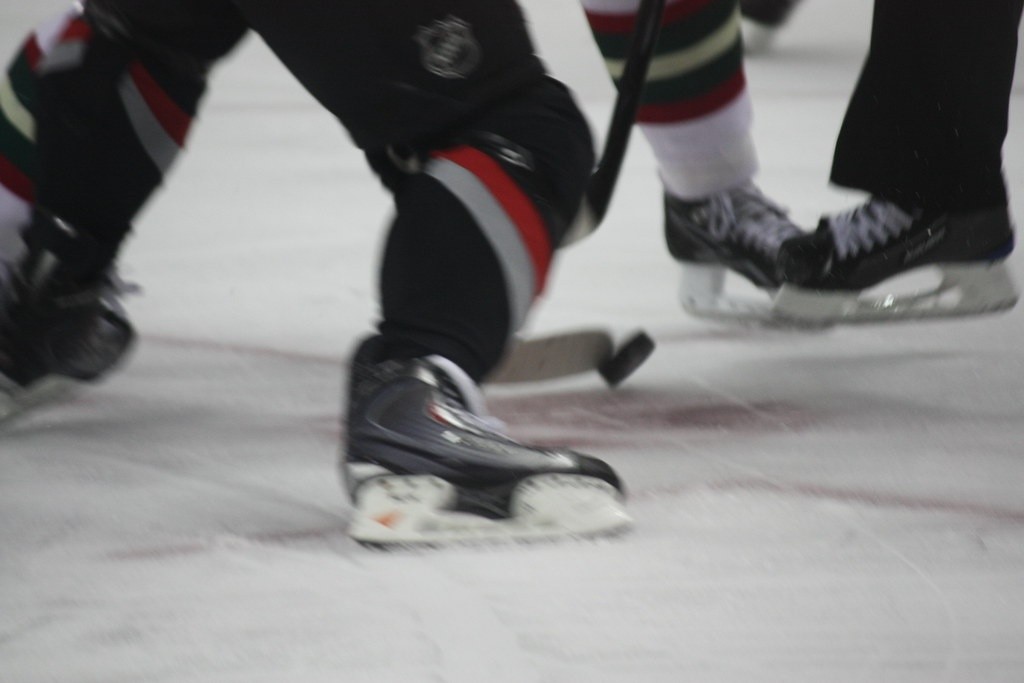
581, 0, 1024, 326
0, 0, 631, 549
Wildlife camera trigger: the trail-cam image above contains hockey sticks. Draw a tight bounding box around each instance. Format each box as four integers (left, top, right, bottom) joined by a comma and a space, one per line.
555, 0, 665, 250
479, 330, 613, 387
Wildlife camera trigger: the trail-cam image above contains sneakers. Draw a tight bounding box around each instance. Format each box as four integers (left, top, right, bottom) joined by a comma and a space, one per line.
773, 200, 1019, 322
342, 346, 632, 548
0, 259, 139, 419
664, 180, 808, 318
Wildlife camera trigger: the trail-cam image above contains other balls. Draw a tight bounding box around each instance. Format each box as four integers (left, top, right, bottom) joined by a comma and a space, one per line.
598, 328, 656, 389
